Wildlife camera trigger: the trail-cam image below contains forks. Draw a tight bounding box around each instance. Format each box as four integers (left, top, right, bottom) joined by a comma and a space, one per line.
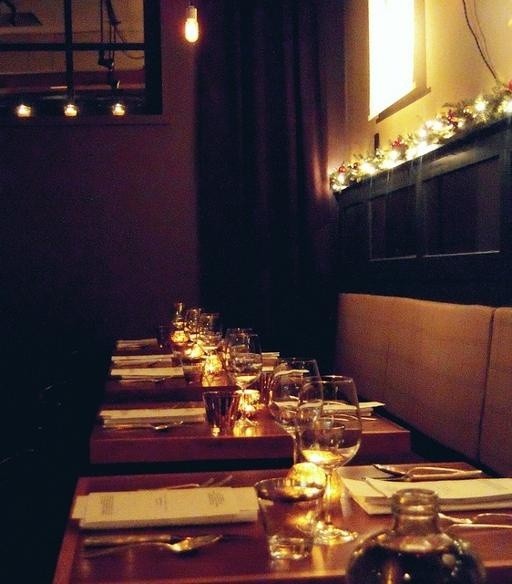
437, 510, 512, 527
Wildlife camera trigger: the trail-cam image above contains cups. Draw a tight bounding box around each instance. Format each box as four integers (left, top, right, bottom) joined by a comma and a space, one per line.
257, 476, 325, 559
202, 391, 240, 433
153, 323, 168, 348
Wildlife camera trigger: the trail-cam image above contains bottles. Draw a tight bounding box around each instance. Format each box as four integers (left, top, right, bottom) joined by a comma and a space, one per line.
347, 489, 489, 582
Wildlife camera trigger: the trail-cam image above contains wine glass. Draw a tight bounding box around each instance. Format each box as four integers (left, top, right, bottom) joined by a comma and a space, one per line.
270, 356, 319, 496
294, 375, 362, 544
169, 300, 263, 419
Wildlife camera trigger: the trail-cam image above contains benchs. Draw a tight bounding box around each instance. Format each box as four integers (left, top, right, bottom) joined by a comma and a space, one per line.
336, 293, 495, 460
478, 306, 511, 473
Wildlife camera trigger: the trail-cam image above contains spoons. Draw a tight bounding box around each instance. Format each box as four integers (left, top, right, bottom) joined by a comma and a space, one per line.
101, 422, 181, 430
119, 376, 165, 383
375, 462, 483, 478
85, 535, 220, 556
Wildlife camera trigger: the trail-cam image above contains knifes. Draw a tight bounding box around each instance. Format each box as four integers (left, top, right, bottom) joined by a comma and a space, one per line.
82, 533, 251, 548
365, 495, 512, 507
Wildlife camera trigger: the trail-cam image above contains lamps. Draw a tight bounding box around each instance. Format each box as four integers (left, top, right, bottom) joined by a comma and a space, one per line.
14, 93, 32, 119
184, 0, 198, 41
62, 96, 79, 118
109, 25, 127, 118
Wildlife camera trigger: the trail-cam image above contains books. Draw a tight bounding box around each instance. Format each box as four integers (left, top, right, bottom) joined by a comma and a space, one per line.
119, 339, 154, 347
262, 352, 279, 359
111, 368, 184, 378
103, 408, 206, 425
112, 354, 172, 365
342, 476, 512, 515
79, 486, 259, 529
323, 401, 373, 417
